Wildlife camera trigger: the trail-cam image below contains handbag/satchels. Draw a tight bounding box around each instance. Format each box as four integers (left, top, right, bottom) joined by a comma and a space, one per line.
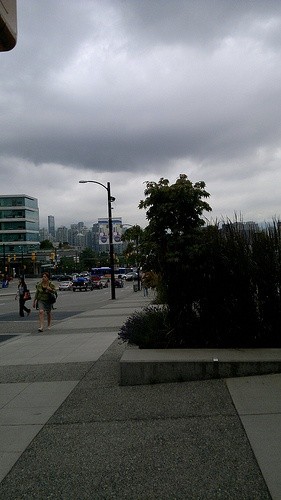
23, 289, 32, 301
48, 283, 57, 304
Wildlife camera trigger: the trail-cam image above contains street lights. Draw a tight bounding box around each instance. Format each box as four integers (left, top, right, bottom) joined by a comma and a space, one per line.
2, 242, 13, 282
78, 180, 115, 300
119, 223, 140, 291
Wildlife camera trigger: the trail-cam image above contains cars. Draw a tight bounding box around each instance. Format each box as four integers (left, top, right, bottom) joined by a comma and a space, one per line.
49, 271, 89, 282
91, 272, 138, 290
59, 281, 73, 291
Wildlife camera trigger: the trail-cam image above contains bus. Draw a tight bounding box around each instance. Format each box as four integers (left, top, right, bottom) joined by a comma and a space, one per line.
89, 267, 134, 279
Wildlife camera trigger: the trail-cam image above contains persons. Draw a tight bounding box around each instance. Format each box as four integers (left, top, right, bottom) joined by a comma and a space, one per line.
33, 272, 58, 332
141, 276, 149, 297
5, 275, 9, 286
15, 275, 31, 318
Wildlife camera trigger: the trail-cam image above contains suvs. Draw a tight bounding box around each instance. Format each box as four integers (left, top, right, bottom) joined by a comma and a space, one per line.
72, 277, 95, 292
